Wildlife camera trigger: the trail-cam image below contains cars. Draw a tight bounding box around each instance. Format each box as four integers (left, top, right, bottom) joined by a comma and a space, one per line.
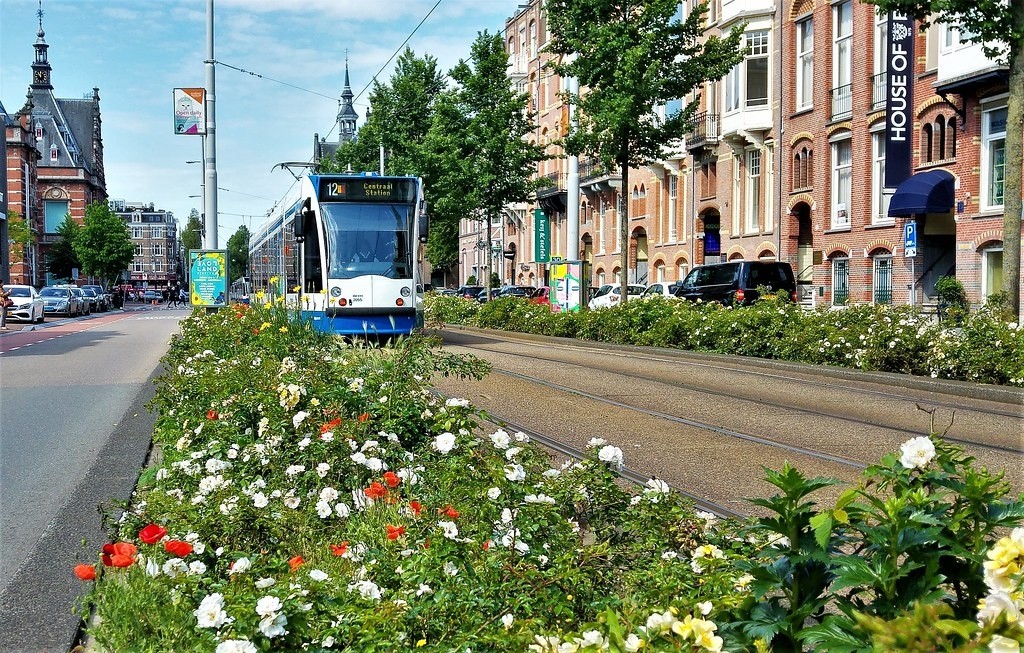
65, 284, 114, 316
586, 282, 645, 312
37, 286, 80, 318
416, 283, 537, 302
3, 285, 46, 324
526, 286, 551, 306
126, 291, 163, 303
178, 292, 189, 302
638, 282, 680, 303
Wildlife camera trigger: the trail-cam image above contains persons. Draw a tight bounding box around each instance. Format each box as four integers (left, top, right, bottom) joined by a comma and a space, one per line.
118, 288, 123, 310
13, 278, 20, 285
176, 287, 188, 307
0, 280, 13, 330
352, 239, 379, 262
165, 287, 178, 308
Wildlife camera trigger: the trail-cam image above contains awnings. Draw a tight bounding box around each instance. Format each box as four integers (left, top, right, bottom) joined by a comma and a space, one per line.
887, 171, 955, 218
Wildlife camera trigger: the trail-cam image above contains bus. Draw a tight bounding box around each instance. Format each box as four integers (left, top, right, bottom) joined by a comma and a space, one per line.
250, 161, 429, 341
230, 276, 250, 305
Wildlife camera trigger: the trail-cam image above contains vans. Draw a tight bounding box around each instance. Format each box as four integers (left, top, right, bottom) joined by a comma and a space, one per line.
674, 260, 799, 310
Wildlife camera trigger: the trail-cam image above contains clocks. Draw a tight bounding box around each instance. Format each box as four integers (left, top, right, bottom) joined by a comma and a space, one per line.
34, 70, 47, 83
